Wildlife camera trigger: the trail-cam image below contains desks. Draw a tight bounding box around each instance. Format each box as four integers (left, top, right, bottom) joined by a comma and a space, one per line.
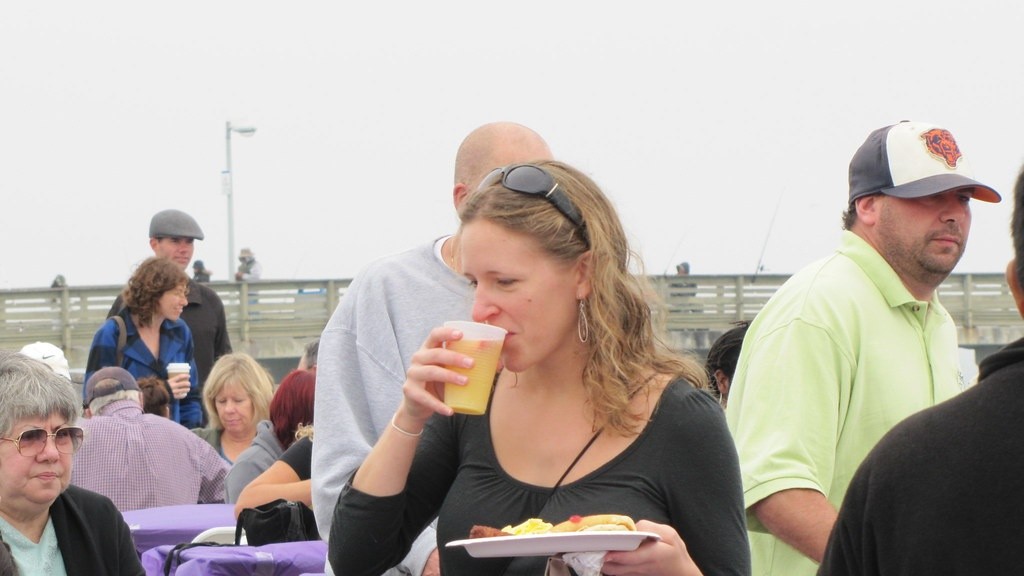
117, 503, 244, 557
140, 540, 326, 574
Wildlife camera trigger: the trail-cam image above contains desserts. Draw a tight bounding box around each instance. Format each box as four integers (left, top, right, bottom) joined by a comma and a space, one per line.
465, 510, 637, 542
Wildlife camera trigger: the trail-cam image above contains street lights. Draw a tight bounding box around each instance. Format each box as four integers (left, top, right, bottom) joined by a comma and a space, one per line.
221, 120, 256, 281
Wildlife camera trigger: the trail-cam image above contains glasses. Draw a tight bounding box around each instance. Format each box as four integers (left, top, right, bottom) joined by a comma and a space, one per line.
0, 425, 87, 457
475, 162, 593, 253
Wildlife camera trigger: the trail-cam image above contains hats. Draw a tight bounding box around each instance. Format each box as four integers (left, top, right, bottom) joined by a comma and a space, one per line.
848, 120, 1004, 205
148, 209, 206, 242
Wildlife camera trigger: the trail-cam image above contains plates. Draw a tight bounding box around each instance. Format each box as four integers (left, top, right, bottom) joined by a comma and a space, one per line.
442, 529, 663, 559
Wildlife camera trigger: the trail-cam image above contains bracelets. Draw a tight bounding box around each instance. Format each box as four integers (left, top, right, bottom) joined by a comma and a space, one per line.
392, 414, 426, 438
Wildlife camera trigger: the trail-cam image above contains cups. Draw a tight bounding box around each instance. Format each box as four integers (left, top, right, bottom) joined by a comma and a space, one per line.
167, 361, 190, 380
435, 318, 508, 420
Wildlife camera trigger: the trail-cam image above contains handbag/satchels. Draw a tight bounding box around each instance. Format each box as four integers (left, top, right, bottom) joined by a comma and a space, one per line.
240, 495, 319, 547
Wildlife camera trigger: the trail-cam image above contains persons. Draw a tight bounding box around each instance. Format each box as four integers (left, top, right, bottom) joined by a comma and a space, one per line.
329, 161, 759, 576
0, 351, 146, 576
48, 276, 69, 329
188, 262, 209, 284
234, 429, 312, 518
83, 255, 204, 429
668, 263, 703, 315
310, 122, 560, 576
297, 341, 319, 373
66, 365, 231, 516
188, 352, 273, 464
224, 371, 315, 503
137, 375, 176, 421
816, 167, 1024, 576
109, 210, 233, 378
724, 119, 1002, 576
709, 320, 749, 412
233, 248, 259, 318
21, 341, 73, 387
